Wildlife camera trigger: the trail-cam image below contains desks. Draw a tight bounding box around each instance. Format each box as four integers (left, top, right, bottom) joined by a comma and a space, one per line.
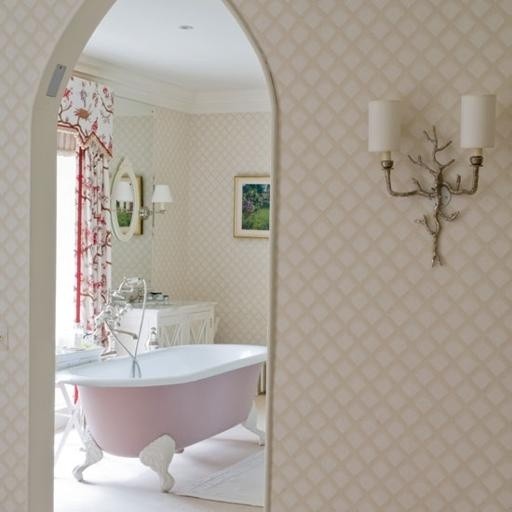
54, 346, 104, 459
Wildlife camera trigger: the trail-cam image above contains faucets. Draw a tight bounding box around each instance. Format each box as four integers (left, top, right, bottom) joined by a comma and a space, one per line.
113, 329, 137, 340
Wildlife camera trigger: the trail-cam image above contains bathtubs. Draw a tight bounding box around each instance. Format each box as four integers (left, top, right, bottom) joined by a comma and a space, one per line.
57, 344, 268, 494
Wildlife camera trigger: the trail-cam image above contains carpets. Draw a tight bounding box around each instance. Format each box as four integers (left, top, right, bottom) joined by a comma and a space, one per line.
175, 447, 265, 507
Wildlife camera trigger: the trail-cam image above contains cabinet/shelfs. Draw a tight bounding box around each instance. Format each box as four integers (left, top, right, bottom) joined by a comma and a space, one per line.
125, 301, 219, 346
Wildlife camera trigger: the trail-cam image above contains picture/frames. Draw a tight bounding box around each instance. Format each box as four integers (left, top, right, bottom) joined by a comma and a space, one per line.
233, 176, 272, 238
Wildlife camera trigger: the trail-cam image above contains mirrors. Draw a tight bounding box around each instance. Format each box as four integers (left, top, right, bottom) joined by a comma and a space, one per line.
110, 157, 140, 242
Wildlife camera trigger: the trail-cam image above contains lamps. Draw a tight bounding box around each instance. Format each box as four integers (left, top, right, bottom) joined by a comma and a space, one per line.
369, 95, 497, 265
116, 181, 133, 211
